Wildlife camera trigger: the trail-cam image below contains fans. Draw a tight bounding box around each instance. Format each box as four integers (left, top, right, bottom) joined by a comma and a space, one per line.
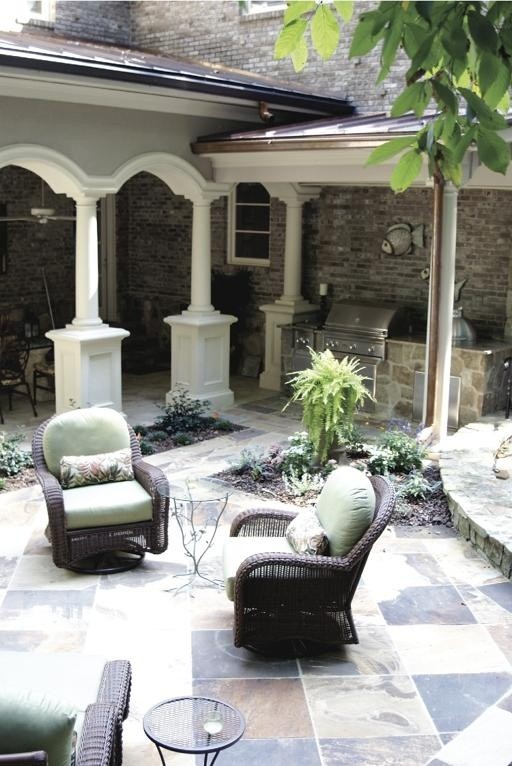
0, 169, 78, 228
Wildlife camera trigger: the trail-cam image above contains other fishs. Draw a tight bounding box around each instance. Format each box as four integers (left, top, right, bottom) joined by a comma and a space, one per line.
379, 222, 425, 257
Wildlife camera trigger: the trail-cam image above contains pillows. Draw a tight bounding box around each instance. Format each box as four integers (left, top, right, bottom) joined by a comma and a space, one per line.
56, 446, 134, 489
281, 507, 333, 558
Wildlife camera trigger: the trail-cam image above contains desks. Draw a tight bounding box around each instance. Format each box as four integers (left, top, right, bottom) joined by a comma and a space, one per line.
157, 476, 232, 594
136, 695, 247, 766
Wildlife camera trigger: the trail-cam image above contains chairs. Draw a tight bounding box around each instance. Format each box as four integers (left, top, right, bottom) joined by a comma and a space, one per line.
0, 655, 140, 766
33, 338, 56, 405
218, 463, 400, 667
0, 332, 39, 423
32, 404, 172, 579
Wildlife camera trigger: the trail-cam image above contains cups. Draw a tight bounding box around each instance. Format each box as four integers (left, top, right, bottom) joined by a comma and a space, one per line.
201, 712, 223, 735
